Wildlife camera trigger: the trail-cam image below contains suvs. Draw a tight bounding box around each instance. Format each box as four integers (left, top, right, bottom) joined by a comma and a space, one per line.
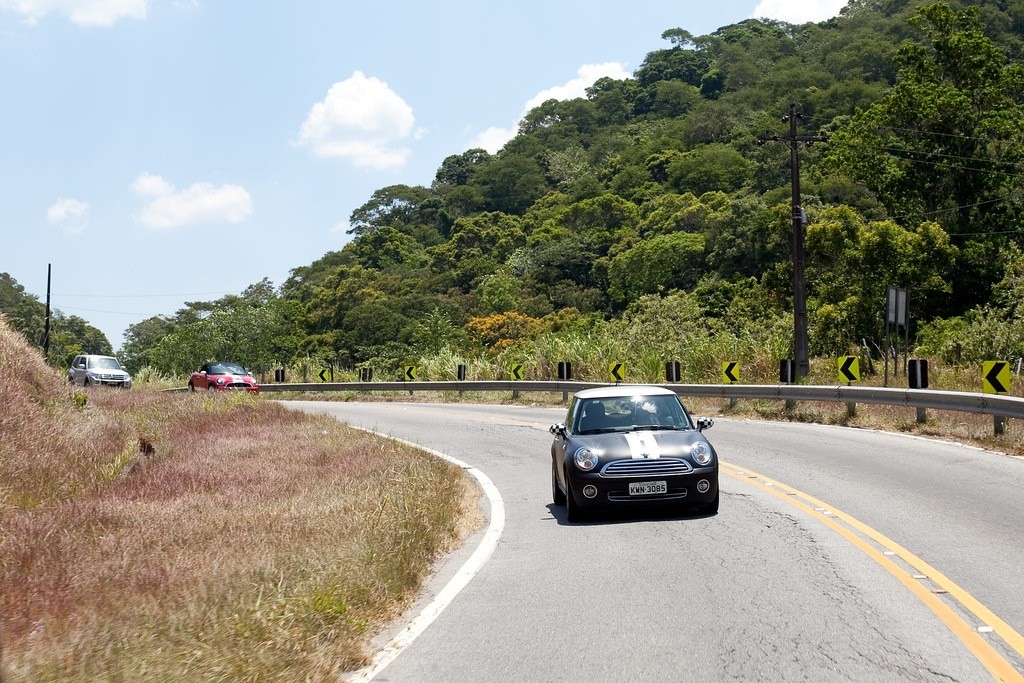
67, 355, 132, 391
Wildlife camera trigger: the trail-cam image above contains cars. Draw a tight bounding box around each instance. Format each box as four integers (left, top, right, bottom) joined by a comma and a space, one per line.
549, 386, 720, 522
187, 362, 260, 396
